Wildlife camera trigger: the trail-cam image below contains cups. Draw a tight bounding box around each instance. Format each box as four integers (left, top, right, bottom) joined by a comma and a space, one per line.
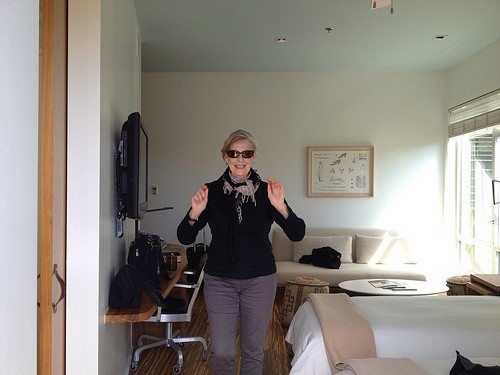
165, 255, 177, 280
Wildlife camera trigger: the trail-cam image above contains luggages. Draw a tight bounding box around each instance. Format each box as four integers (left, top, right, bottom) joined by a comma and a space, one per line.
186, 227, 210, 291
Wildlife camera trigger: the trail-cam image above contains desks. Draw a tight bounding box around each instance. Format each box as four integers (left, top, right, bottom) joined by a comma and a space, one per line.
106, 244, 187, 325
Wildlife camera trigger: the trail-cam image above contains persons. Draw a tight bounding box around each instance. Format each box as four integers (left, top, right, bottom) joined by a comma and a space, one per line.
176, 129, 306, 375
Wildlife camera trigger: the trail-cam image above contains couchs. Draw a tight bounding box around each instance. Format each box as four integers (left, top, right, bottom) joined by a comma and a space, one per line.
272, 228, 427, 288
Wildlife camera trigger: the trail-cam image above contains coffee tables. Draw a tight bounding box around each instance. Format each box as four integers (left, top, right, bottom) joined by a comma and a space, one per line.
338, 278, 450, 296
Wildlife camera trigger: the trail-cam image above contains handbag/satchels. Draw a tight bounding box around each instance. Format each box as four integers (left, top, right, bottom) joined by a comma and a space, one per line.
299, 246, 342, 269
110, 233, 166, 309
449, 350, 500, 375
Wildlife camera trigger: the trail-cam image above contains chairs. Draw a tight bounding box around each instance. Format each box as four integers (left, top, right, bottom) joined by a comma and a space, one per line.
130, 253, 208, 374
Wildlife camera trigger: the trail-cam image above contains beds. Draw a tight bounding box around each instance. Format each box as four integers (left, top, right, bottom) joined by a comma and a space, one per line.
285, 297, 500, 375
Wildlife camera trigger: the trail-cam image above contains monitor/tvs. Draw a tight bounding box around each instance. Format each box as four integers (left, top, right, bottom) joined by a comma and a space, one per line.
116, 112, 149, 221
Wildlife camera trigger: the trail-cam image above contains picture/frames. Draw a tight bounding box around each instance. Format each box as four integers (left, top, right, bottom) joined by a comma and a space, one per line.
307, 146, 375, 197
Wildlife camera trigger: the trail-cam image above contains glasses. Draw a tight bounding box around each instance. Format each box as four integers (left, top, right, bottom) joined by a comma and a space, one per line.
226, 150, 256, 159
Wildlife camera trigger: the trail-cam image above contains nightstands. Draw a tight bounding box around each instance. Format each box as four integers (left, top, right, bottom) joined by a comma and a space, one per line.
466, 274, 500, 295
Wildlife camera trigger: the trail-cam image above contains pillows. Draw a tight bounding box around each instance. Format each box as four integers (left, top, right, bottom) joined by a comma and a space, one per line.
292, 235, 353, 263
355, 233, 416, 264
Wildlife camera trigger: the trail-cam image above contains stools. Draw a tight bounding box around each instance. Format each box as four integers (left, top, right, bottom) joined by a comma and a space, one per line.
283, 277, 329, 328
446, 275, 469, 296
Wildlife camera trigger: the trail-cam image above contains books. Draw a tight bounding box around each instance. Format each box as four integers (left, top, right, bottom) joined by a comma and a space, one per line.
368, 280, 417, 291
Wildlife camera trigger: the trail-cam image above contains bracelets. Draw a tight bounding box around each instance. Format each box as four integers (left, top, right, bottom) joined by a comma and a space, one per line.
188, 215, 198, 221
